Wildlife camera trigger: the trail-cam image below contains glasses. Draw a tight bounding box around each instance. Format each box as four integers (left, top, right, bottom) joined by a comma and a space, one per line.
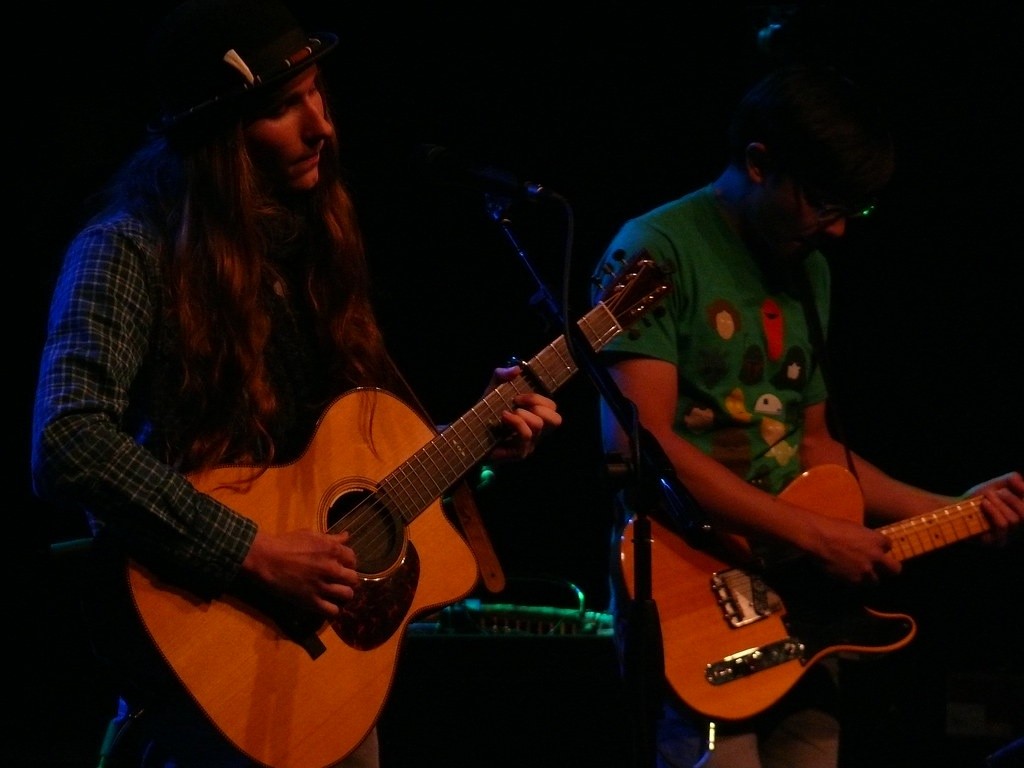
805, 186, 882, 224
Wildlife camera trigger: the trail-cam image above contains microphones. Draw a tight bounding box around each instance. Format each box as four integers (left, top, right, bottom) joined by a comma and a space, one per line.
426, 148, 568, 205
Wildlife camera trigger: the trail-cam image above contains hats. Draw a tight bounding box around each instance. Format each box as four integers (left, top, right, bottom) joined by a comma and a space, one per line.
163, 21, 340, 158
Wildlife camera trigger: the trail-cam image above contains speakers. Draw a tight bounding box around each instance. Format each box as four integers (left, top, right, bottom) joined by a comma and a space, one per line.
376, 613, 659, 768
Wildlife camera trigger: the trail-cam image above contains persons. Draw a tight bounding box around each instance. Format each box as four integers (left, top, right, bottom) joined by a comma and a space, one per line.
33, 25, 561, 768
593, 74, 1024, 768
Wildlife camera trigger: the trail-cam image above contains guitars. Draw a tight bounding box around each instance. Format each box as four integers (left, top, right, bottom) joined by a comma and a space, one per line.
92, 245, 681, 768
597, 437, 1024, 732
